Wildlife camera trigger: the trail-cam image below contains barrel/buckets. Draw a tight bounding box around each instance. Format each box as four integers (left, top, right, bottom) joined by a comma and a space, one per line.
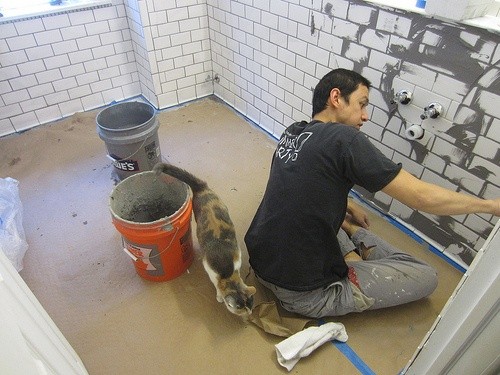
108, 171, 193, 283
95, 101, 163, 181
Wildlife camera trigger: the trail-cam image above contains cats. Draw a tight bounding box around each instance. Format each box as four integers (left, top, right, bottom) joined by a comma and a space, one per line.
152, 162, 256, 318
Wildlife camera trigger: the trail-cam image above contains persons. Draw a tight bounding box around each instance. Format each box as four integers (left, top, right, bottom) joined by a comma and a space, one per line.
244, 68, 500, 319
